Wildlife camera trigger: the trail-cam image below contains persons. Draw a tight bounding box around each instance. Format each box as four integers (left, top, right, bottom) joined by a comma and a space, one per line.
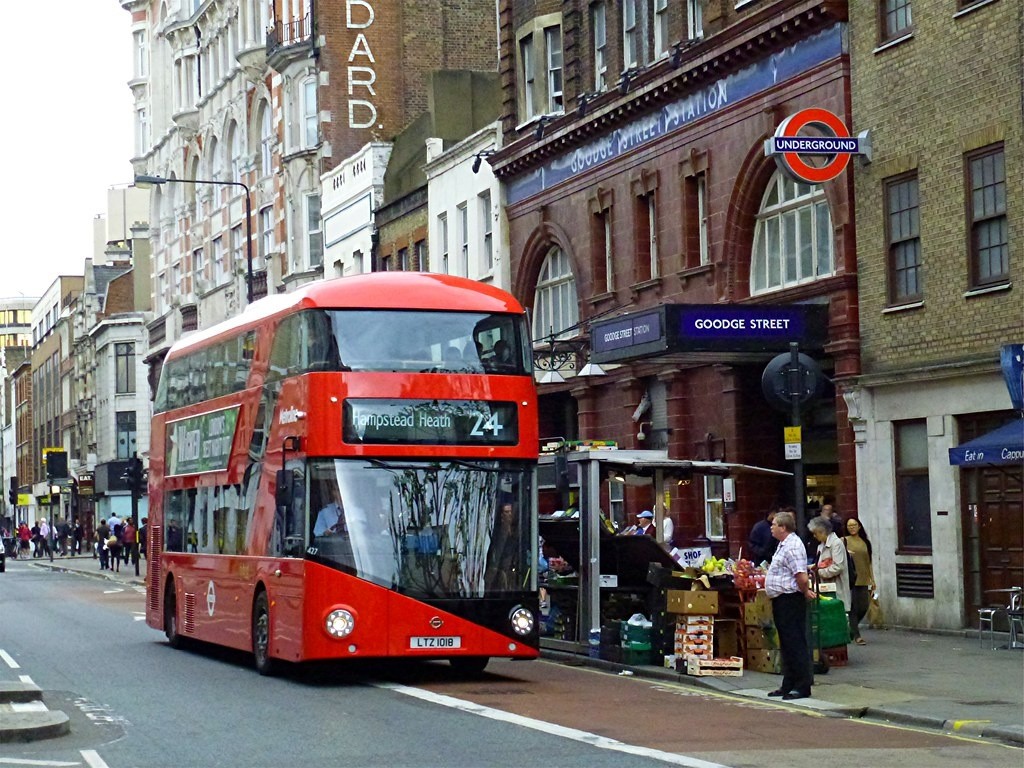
92, 519, 111, 570
106, 511, 121, 535
764, 512, 817, 700
314, 484, 367, 535
1, 526, 9, 537
72, 519, 84, 556
501, 503, 513, 533
54, 517, 72, 556
168, 519, 182, 552
47, 521, 58, 556
38, 517, 50, 557
360, 337, 513, 366
636, 504, 674, 545
110, 524, 123, 572
138, 517, 147, 561
30, 521, 43, 558
16, 521, 32, 559
121, 517, 136, 565
747, 504, 877, 645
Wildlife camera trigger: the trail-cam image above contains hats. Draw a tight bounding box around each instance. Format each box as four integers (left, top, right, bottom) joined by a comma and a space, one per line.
636, 510, 653, 518
20, 521, 25, 524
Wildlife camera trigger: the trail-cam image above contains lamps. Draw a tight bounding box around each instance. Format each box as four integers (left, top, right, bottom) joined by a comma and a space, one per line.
618, 65, 645, 93
534, 113, 563, 139
577, 88, 604, 116
670, 35, 701, 69
636, 421, 652, 439
471, 148, 497, 172
538, 326, 606, 385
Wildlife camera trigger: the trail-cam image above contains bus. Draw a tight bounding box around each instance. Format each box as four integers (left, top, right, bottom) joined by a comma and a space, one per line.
146, 272, 572, 675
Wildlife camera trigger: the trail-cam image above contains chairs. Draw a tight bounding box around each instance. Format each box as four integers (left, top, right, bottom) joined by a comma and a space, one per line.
977, 592, 1023, 650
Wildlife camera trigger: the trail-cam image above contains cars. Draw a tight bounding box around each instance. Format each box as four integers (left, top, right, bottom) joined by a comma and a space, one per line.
0, 535, 5, 572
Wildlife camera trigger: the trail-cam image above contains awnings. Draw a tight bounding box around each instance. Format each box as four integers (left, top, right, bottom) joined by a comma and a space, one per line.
948, 413, 1024, 466
588, 304, 830, 363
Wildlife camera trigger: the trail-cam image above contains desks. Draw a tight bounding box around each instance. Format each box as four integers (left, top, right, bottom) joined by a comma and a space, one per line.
986, 587, 1023, 649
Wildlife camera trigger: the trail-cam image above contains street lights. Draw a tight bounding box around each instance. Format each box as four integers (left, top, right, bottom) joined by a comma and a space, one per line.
134, 175, 253, 304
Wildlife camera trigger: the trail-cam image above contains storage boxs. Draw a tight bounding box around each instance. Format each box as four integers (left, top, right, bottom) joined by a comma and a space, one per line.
741, 587, 781, 673
665, 588, 718, 660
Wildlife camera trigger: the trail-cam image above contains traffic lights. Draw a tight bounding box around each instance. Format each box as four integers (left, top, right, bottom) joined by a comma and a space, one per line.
121, 463, 147, 493
9, 476, 19, 504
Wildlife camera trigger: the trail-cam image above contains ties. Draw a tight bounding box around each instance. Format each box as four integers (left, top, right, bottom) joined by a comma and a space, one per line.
337, 512, 345, 535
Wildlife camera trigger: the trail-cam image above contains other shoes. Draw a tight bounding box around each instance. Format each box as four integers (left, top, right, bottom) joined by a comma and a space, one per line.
782, 690, 811, 700
767, 686, 792, 697
855, 638, 866, 645
100, 566, 120, 573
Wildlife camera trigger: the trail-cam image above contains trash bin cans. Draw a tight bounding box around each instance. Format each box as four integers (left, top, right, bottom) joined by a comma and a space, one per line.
2, 537, 16, 556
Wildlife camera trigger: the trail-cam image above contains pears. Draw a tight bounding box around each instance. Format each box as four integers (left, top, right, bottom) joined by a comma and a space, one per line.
701, 555, 727, 571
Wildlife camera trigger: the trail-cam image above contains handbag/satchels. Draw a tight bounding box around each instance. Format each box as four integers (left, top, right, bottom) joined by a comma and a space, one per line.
844, 538, 857, 589
108, 535, 118, 547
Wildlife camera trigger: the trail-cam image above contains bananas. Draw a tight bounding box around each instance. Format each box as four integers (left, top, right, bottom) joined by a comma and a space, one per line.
818, 594, 833, 600
